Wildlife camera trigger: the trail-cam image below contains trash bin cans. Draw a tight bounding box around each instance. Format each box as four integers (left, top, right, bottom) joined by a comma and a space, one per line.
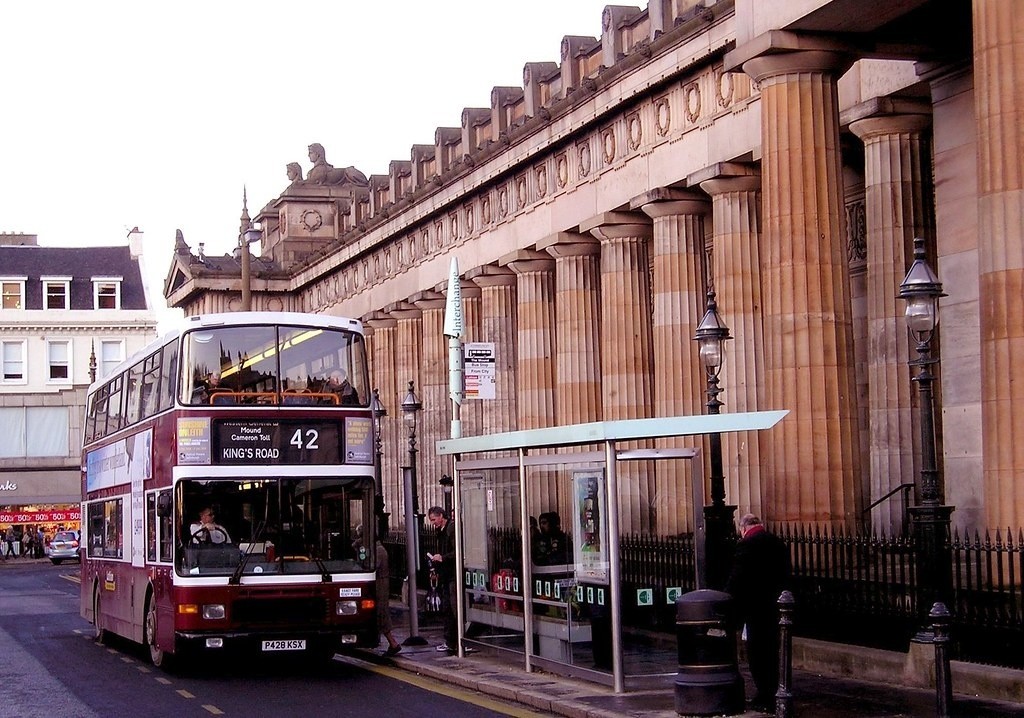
672, 588, 746, 716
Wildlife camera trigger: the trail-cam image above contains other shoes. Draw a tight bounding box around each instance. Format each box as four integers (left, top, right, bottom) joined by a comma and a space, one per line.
5, 557, 10, 559
750, 699, 777, 714
382, 643, 402, 657
22, 555, 26, 558
15, 555, 19, 559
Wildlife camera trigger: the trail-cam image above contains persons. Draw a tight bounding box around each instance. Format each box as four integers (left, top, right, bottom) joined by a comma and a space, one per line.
0, 372, 623, 672
726, 514, 793, 715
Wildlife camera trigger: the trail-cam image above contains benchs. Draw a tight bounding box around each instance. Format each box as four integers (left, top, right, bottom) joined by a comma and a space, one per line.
463, 588, 592, 665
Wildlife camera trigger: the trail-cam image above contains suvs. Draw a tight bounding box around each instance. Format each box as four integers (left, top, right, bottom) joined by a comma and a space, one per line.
49, 530, 81, 565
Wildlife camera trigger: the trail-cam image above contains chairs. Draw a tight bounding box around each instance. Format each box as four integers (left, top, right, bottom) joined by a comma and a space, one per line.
258, 388, 313, 405
208, 388, 237, 406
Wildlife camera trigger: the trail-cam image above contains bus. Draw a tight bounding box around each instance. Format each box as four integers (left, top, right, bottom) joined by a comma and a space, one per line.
81, 312, 382, 676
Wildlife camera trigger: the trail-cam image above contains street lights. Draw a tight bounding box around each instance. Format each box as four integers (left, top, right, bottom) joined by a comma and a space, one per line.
892, 236, 955, 645
692, 276, 744, 633
396, 379, 432, 586
366, 387, 391, 580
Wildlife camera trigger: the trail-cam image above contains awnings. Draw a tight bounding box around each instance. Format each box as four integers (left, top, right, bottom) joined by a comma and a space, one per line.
0, 471, 82, 505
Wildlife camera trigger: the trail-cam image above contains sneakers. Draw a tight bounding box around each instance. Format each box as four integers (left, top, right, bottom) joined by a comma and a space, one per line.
436, 641, 457, 651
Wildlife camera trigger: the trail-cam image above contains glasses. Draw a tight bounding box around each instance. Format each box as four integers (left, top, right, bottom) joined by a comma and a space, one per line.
203, 512, 215, 517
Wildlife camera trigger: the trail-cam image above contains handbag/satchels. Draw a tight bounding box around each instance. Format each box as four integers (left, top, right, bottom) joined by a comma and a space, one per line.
22, 533, 32, 544
6, 535, 15, 541
